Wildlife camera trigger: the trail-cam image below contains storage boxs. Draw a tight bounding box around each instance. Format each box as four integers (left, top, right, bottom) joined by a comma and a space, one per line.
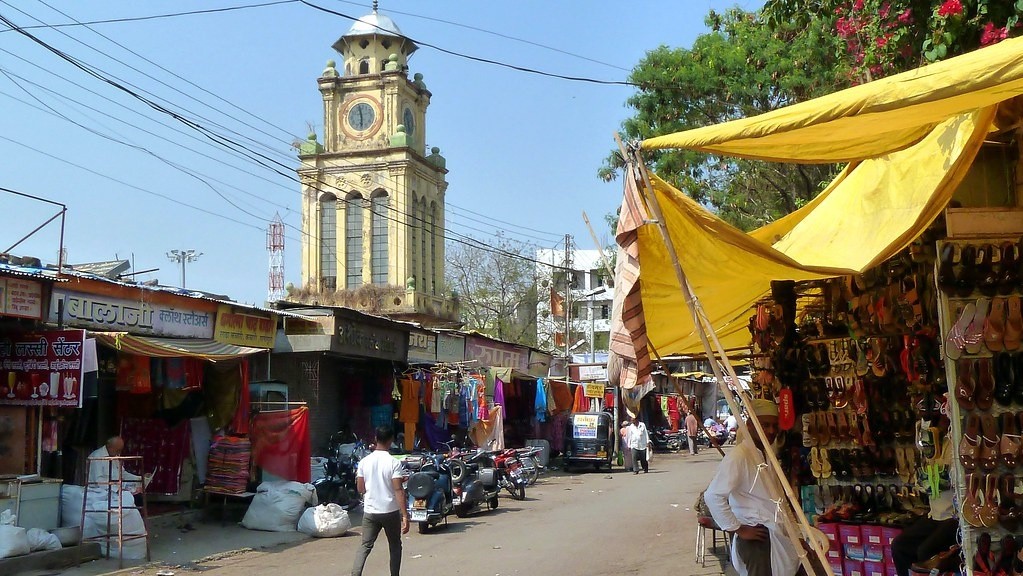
0, 473, 63, 532
817, 522, 901, 576
801, 487, 816, 526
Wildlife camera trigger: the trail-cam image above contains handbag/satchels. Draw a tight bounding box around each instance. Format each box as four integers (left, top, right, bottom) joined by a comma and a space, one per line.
618, 451, 623, 466
646, 447, 653, 462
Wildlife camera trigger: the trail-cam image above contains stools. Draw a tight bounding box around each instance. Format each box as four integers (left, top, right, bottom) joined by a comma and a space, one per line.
76, 456, 150, 569
697, 518, 730, 568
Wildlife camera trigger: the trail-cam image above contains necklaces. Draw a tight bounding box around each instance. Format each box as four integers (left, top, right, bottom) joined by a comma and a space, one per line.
376, 449, 385, 450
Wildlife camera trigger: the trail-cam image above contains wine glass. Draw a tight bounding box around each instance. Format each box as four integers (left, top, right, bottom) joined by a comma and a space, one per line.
7, 372, 15, 397
31, 373, 39, 398
63, 378, 77, 399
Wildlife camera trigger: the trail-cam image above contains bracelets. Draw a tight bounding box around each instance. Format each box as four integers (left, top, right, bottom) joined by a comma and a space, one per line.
402, 515, 408, 519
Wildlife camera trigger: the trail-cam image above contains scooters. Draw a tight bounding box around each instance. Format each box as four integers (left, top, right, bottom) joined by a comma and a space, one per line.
311, 429, 544, 533
649, 424, 738, 452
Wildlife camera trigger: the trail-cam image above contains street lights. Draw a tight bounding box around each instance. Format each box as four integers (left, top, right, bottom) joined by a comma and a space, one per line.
564, 283, 608, 392
166, 248, 204, 289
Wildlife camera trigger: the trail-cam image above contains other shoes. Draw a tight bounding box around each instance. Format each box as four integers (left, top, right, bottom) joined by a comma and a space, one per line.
644, 471, 648, 473
633, 471, 638, 475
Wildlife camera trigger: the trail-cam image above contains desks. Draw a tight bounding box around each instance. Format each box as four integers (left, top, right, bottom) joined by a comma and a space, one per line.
197, 489, 256, 528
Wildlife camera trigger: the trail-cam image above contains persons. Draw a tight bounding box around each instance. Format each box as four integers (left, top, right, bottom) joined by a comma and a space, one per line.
685, 409, 698, 454
723, 413, 738, 429
89, 436, 152, 515
350, 425, 410, 576
891, 427, 960, 576
704, 416, 718, 447
704, 399, 815, 576
620, 417, 650, 475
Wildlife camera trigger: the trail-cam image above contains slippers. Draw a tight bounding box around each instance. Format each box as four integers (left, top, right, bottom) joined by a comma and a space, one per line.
748, 238, 1023, 576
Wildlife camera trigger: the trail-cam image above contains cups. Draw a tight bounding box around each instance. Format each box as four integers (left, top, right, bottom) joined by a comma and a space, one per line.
50, 372, 60, 398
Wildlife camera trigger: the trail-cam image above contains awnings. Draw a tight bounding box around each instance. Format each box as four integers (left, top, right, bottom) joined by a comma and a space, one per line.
608, 33, 1023, 576
87, 329, 270, 364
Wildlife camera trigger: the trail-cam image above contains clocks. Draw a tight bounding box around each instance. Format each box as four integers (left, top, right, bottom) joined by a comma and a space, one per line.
401, 102, 417, 136
339, 95, 384, 141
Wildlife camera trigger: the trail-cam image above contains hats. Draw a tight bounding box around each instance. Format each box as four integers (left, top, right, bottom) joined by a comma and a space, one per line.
750, 399, 778, 416
621, 421, 629, 426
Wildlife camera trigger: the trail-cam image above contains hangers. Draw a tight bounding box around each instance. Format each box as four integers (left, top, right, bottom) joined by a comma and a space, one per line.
402, 364, 421, 376
430, 363, 454, 383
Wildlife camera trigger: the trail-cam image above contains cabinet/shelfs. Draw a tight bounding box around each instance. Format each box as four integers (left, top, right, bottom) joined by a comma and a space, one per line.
934, 239, 1022, 576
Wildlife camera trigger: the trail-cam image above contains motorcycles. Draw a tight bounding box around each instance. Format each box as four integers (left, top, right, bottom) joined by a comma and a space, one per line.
560, 410, 616, 470
